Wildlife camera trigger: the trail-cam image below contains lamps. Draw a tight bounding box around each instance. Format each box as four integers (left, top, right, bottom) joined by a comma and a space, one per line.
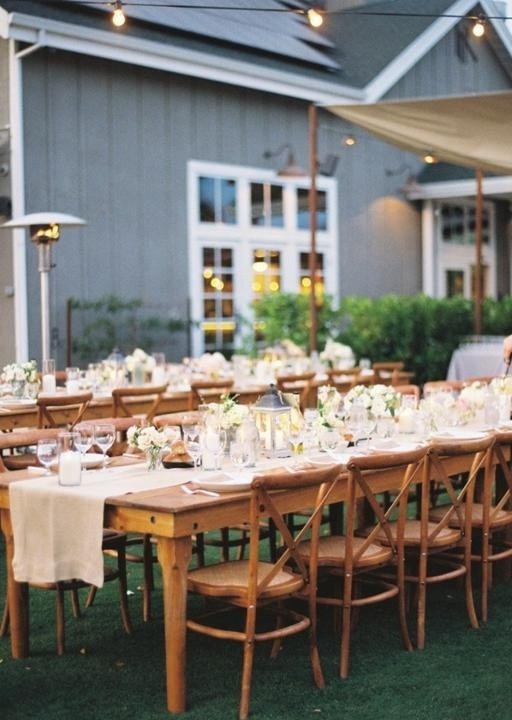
308, 8, 322, 27
471, 14, 487, 37
347, 137, 355, 147
204, 256, 313, 294
110, 0, 128, 28
425, 154, 435, 164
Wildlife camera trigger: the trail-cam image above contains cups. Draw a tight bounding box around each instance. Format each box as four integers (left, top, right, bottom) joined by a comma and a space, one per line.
40, 359, 165, 391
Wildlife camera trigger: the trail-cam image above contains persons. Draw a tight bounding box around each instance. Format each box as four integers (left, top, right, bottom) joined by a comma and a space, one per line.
503, 335, 512, 364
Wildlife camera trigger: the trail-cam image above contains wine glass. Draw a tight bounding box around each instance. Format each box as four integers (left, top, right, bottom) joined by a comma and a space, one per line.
36, 380, 511, 477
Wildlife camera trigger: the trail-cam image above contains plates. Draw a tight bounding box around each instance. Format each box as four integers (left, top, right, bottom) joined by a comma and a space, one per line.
55, 427, 494, 493
